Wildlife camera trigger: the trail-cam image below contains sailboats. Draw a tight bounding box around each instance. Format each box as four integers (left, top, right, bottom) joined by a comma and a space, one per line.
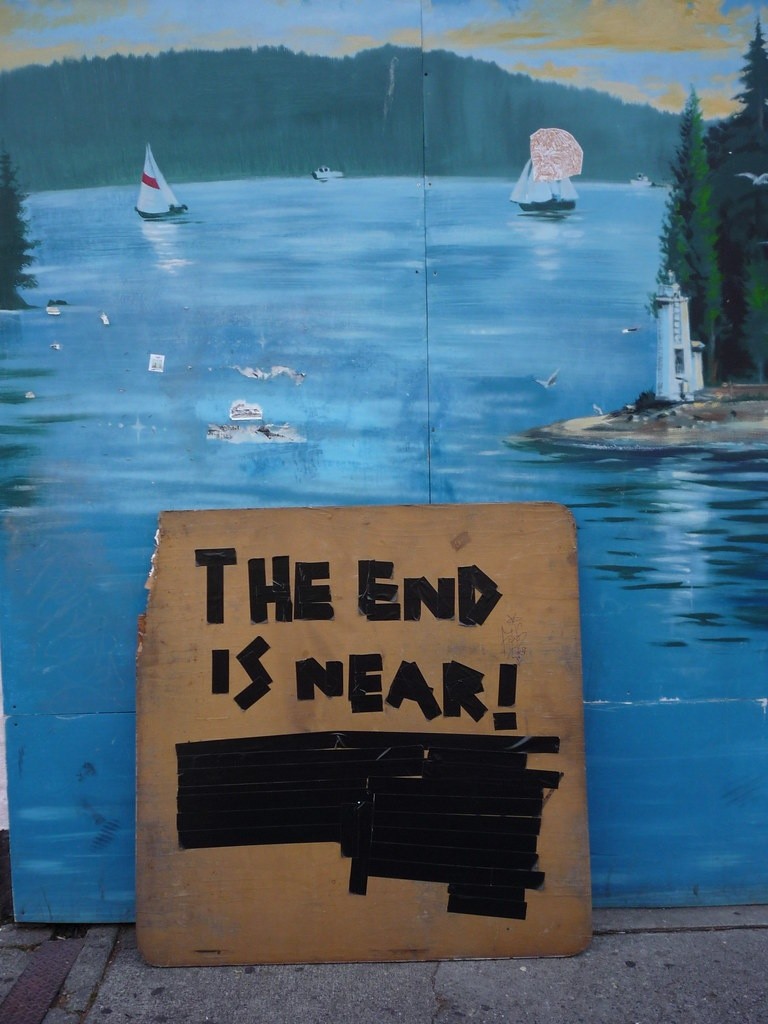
133, 141, 188, 219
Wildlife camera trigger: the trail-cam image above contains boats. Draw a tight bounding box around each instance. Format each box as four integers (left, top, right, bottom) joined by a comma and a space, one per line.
310, 166, 344, 180
630, 176, 654, 187
507, 156, 581, 212
